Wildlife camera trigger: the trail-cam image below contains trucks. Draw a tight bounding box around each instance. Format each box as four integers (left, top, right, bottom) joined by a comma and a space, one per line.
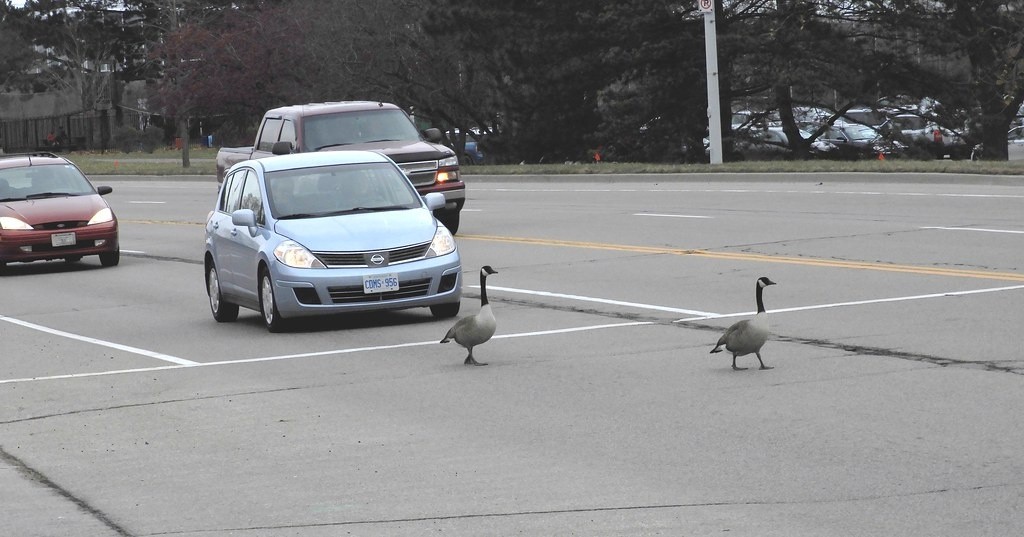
216, 100, 466, 237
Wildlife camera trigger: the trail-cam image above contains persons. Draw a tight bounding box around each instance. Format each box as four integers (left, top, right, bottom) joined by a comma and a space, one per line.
347, 172, 384, 208
0, 178, 13, 198
269, 177, 307, 218
47, 127, 59, 146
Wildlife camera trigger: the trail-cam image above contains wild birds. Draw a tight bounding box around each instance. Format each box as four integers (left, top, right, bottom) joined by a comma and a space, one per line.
440, 265, 498, 366
709, 277, 777, 371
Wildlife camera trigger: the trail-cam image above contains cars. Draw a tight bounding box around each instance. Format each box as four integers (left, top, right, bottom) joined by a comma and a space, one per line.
637, 89, 1024, 161
0, 152, 121, 275
439, 125, 495, 165
201, 150, 463, 334
